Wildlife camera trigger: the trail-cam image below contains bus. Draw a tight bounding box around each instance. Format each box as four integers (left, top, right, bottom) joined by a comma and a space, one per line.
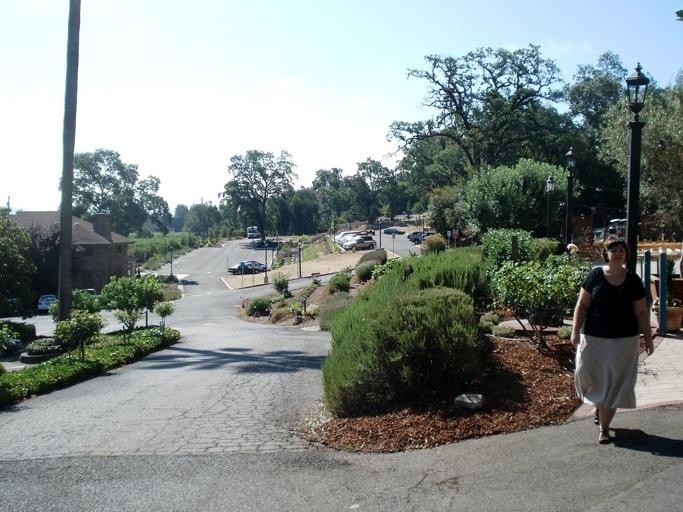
246, 226, 257, 239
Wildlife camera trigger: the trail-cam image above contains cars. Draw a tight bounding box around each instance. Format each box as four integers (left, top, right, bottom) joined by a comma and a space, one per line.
375, 215, 391, 222
74, 289, 96, 296
384, 227, 405, 235
227, 260, 266, 274
35, 294, 59, 311
406, 231, 435, 245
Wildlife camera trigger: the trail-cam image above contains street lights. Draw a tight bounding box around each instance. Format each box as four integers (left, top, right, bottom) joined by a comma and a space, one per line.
558, 201, 565, 234
544, 174, 554, 238
563, 145, 576, 264
624, 61, 649, 274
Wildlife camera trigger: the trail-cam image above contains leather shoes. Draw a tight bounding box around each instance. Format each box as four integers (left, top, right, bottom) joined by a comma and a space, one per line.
599, 428, 609, 444
594, 411, 599, 423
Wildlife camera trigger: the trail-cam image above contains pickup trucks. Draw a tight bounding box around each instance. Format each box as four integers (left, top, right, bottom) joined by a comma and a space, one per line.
334, 228, 376, 251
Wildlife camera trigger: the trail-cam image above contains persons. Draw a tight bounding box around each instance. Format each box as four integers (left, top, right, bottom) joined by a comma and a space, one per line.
570, 239, 655, 444
